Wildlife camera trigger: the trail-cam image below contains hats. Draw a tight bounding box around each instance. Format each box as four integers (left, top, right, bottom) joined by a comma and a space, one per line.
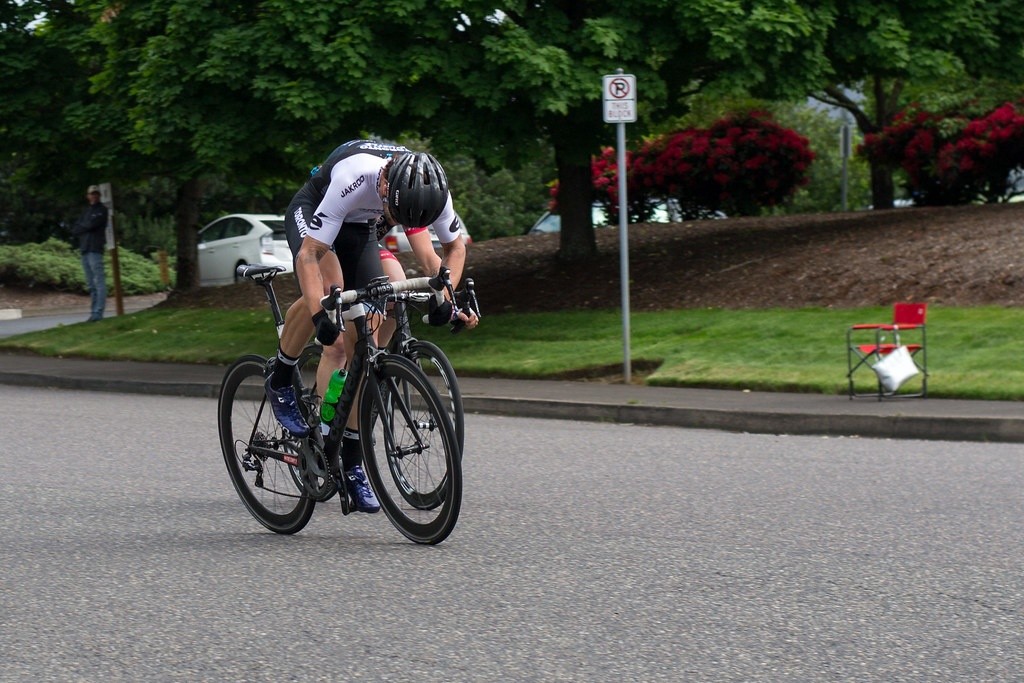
87, 185, 102, 194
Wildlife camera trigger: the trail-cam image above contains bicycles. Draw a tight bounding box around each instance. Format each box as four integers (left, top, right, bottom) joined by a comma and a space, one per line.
217, 263, 480, 546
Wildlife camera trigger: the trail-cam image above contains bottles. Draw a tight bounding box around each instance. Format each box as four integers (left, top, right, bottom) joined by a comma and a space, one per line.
320, 368, 349, 424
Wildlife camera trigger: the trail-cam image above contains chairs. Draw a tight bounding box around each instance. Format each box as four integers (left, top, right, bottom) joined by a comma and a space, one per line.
845, 302, 929, 401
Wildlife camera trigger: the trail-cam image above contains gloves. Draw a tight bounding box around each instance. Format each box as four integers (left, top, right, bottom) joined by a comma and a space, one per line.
428, 293, 452, 326
312, 309, 345, 346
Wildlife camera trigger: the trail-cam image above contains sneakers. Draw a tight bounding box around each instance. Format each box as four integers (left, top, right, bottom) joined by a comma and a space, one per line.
264, 371, 310, 439
343, 466, 381, 514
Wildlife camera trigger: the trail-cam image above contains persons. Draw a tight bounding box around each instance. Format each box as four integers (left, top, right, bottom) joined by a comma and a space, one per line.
72, 185, 109, 322
264, 139, 479, 513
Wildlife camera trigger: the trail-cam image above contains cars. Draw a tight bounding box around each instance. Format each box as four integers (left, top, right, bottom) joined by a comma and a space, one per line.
196, 214, 295, 287
529, 198, 677, 235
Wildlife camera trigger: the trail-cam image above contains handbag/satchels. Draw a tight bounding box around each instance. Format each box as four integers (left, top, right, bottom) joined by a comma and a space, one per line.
872, 325, 919, 392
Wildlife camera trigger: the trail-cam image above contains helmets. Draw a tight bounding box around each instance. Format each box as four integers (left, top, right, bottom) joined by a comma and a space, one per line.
388, 152, 448, 228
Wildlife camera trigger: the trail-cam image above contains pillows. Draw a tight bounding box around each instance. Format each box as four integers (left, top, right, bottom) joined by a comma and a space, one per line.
871, 343, 918, 393
